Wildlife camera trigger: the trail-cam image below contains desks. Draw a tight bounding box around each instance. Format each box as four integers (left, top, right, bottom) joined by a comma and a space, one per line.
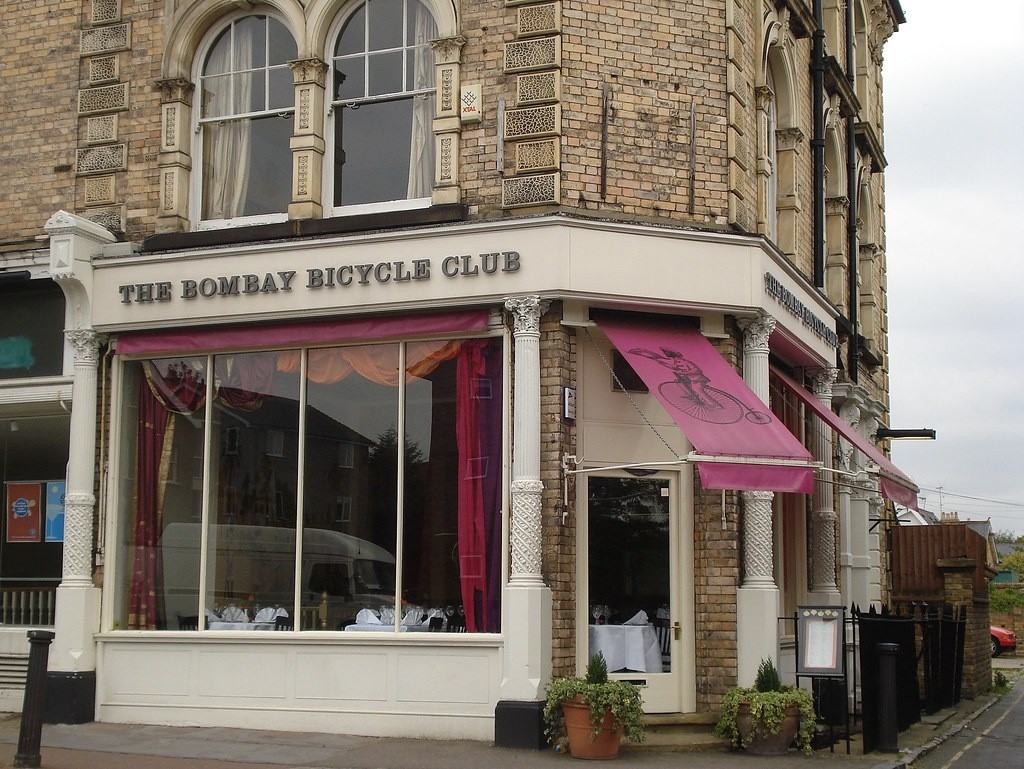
588, 624, 663, 673
345, 624, 446, 632
208, 622, 292, 631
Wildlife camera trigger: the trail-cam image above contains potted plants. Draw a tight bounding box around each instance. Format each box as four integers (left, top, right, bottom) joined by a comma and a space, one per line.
542, 649, 647, 760
711, 655, 815, 758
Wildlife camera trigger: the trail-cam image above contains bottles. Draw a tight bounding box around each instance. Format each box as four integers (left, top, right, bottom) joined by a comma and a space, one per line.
243, 609, 249, 623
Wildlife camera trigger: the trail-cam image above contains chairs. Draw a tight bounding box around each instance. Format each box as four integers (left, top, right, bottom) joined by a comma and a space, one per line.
274, 616, 305, 631
430, 617, 444, 631
337, 618, 356, 631
176, 614, 208, 630
446, 615, 468, 633
649, 617, 670, 672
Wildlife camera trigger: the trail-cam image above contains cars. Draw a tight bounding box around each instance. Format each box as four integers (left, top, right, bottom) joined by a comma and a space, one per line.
990, 625, 1016, 659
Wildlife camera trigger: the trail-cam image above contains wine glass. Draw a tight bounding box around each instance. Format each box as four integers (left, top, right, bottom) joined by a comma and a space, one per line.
592, 605, 612, 625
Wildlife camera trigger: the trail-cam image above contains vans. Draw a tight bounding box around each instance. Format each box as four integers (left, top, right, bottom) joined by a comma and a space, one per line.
162, 522, 427, 630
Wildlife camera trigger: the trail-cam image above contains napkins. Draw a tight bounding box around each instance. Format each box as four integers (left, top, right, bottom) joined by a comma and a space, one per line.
204, 607, 289, 622
356, 607, 447, 625
